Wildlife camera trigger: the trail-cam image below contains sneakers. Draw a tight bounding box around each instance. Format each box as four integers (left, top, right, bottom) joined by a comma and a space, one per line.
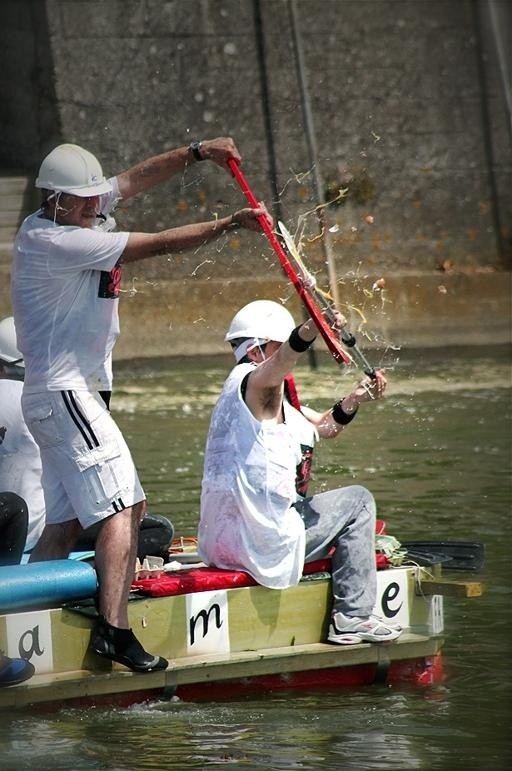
327, 608, 403, 644
88, 617, 168, 670
0, 655, 34, 687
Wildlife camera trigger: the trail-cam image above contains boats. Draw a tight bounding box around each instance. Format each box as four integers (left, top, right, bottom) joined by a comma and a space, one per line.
0, 534, 490, 716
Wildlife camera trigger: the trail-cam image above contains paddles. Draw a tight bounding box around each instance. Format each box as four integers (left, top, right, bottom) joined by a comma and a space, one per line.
276, 221, 377, 378
229, 161, 349, 366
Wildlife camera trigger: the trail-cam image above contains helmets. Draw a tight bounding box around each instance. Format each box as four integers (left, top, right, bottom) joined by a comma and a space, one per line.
0, 317, 26, 367
225, 300, 295, 344
37, 144, 113, 199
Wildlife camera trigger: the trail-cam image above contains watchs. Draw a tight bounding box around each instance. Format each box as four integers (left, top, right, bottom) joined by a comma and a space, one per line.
189, 139, 205, 163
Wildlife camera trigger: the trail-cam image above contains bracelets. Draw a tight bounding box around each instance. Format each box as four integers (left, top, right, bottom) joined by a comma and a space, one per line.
231, 213, 243, 231
287, 323, 318, 355
331, 395, 360, 428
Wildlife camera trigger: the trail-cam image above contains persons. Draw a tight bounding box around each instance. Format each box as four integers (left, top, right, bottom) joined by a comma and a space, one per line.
11, 135, 279, 679
193, 298, 406, 647
1, 313, 44, 567
